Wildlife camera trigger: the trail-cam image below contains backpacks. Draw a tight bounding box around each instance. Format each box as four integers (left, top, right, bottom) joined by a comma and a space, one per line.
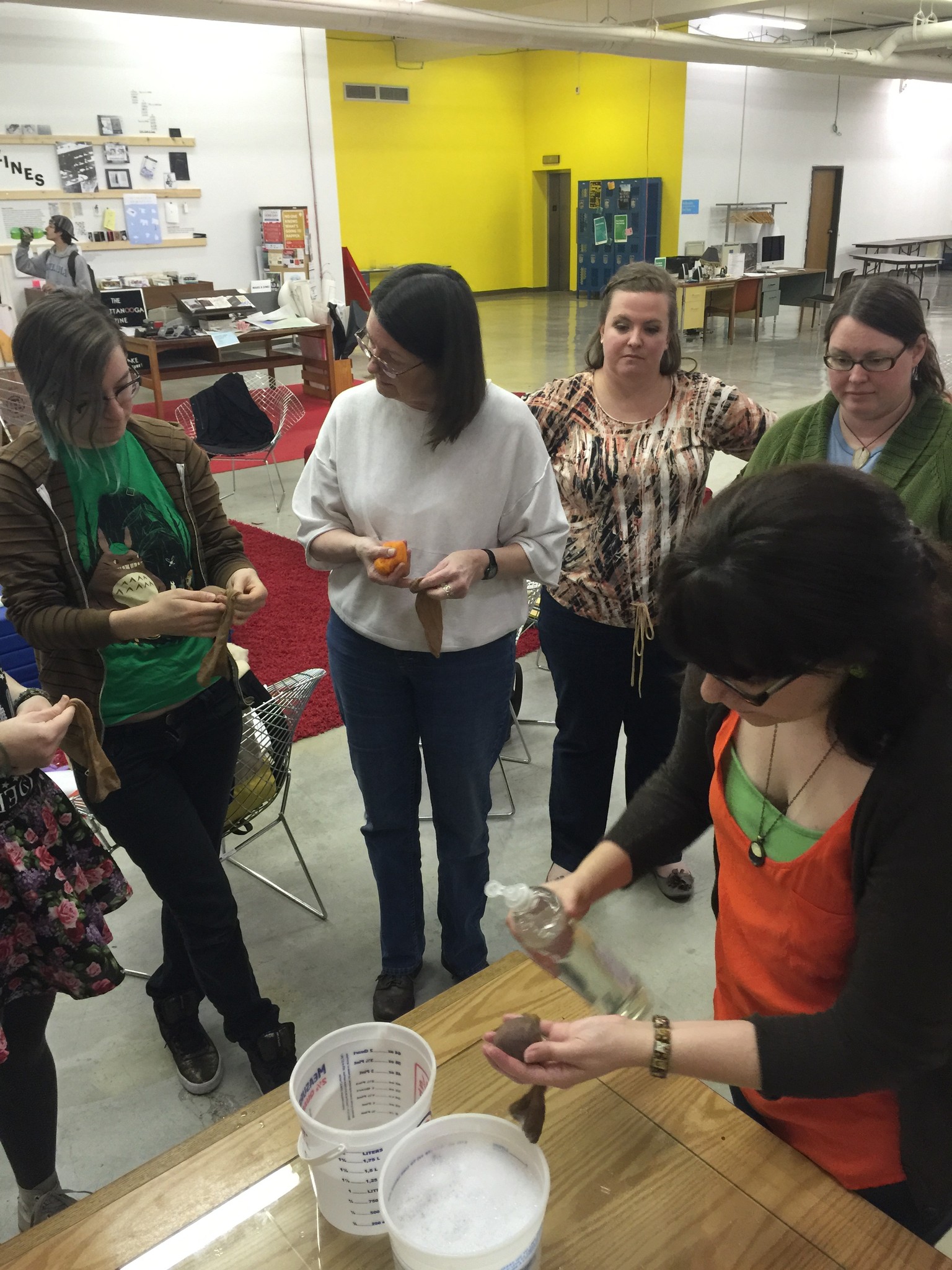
46, 251, 101, 304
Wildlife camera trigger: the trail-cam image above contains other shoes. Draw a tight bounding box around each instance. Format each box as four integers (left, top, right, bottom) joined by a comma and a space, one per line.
653, 868, 695, 898
17, 1169, 78, 1232
246, 1022, 298, 1095
373, 956, 423, 1023
153, 986, 223, 1095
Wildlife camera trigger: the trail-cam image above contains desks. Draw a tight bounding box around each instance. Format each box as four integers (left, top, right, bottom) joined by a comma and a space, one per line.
850, 253, 945, 309
753, 266, 827, 326
359, 265, 452, 291
119, 319, 338, 419
852, 240, 927, 286
172, 289, 257, 327
1, 945, 952, 1269
896, 234, 952, 278
664, 272, 760, 342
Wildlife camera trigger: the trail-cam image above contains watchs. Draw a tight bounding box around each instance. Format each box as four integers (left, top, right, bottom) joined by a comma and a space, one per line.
481, 548, 498, 580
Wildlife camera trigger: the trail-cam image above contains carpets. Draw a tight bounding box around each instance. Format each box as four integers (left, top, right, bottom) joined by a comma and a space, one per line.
132, 380, 367, 474
222, 519, 545, 745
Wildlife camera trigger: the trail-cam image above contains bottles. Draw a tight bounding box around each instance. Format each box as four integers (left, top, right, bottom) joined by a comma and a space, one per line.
483, 878, 674, 1024
11, 226, 47, 239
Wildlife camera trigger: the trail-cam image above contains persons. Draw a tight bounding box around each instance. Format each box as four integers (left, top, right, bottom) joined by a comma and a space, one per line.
740, 276, 952, 549
1, 288, 299, 1096
16, 215, 101, 302
13, 687, 54, 715
525, 261, 780, 904
291, 261, 568, 1022
0, 668, 135, 1234
482, 464, 951, 1248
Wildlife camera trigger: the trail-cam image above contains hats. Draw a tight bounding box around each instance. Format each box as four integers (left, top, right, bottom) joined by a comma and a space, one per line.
49, 215, 78, 242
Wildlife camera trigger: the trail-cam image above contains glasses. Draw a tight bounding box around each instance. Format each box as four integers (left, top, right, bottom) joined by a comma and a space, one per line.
63, 360, 142, 419
823, 342, 910, 372
354, 325, 426, 379
712, 653, 829, 707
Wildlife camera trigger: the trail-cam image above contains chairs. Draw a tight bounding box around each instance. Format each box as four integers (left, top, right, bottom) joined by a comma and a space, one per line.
175, 370, 305, 513
70, 667, 329, 978
703, 277, 762, 346
797, 268, 858, 332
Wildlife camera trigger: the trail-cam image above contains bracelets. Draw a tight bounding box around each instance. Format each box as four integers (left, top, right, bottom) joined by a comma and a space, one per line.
650, 1015, 671, 1079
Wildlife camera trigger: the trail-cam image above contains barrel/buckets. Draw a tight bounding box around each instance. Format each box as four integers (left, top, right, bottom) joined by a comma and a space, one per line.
378, 1113, 549, 1270
288, 1022, 436, 1235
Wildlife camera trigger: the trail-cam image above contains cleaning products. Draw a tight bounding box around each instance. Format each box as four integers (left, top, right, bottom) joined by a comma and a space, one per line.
484, 879, 659, 1024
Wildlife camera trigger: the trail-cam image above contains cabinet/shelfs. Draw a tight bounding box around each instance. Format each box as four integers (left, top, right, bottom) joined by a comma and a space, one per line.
24, 280, 214, 313
576, 177, 662, 299
